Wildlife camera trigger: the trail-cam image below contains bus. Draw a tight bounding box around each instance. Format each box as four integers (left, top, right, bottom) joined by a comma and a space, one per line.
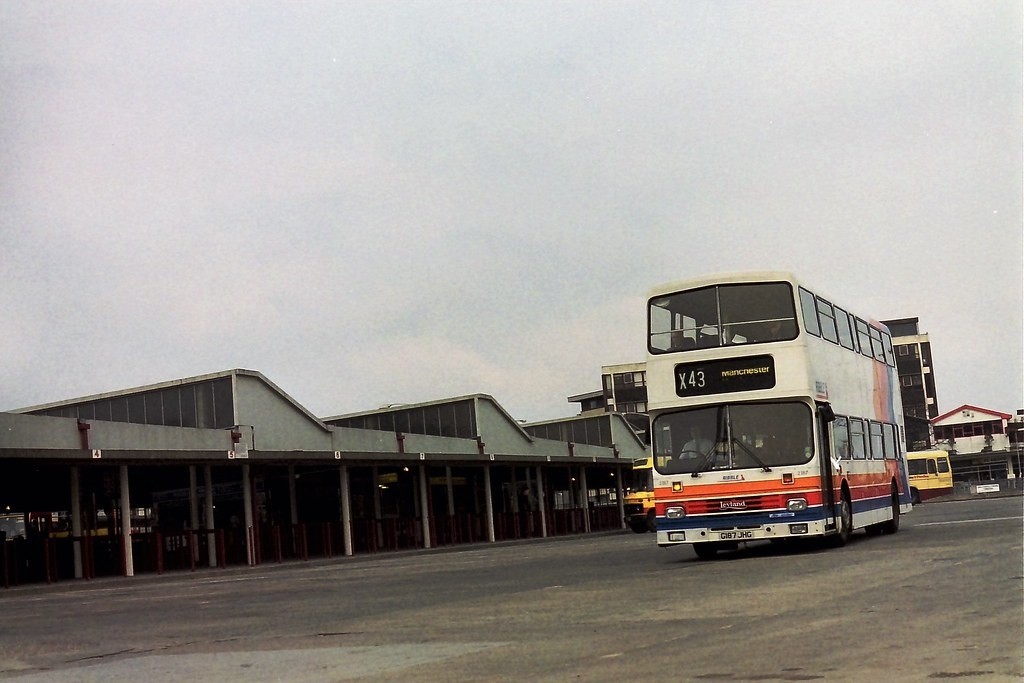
907, 450, 955, 507
619, 454, 672, 534
642, 271, 914, 562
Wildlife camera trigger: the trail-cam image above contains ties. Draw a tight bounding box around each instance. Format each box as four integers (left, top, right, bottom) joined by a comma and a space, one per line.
694, 439, 701, 457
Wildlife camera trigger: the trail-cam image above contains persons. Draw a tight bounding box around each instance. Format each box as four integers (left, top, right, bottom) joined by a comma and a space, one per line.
678, 422, 715, 463
725, 435, 800, 469
668, 331, 689, 352
